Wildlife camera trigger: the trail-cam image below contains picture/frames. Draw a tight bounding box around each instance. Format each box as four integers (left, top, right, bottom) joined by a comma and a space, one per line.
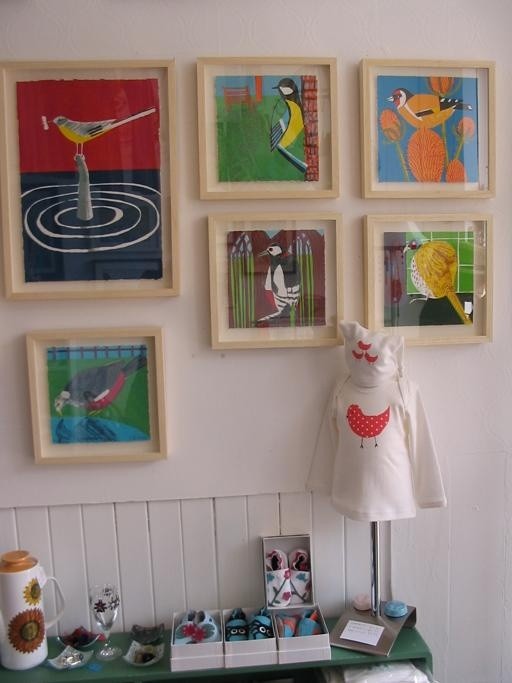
196, 60, 340, 200
26, 325, 167, 461
357, 59, 497, 199
0, 57, 181, 302
1, 614, 433, 683
207, 212, 345, 349
363, 213, 495, 348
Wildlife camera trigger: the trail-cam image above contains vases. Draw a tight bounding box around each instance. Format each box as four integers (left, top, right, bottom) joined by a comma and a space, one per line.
10, 578, 44, 652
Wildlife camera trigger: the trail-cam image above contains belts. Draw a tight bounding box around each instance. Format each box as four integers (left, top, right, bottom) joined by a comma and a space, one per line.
88, 583, 124, 662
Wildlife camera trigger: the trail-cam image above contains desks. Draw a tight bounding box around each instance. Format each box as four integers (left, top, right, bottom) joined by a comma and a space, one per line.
1, 614, 433, 683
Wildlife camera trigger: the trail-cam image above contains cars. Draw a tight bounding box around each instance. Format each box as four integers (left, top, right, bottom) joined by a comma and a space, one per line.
0, 549, 68, 671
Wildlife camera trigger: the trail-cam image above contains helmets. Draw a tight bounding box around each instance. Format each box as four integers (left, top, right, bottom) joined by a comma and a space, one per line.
47, 645, 95, 671
122, 623, 165, 668
58, 626, 101, 649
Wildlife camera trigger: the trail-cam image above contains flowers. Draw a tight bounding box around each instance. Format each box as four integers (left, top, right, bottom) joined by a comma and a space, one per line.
10, 578, 44, 652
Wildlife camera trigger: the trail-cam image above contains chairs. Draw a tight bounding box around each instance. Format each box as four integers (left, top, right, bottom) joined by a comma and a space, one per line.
227, 608, 272, 641
276, 609, 320, 637
266, 549, 311, 606
174, 609, 220, 644
